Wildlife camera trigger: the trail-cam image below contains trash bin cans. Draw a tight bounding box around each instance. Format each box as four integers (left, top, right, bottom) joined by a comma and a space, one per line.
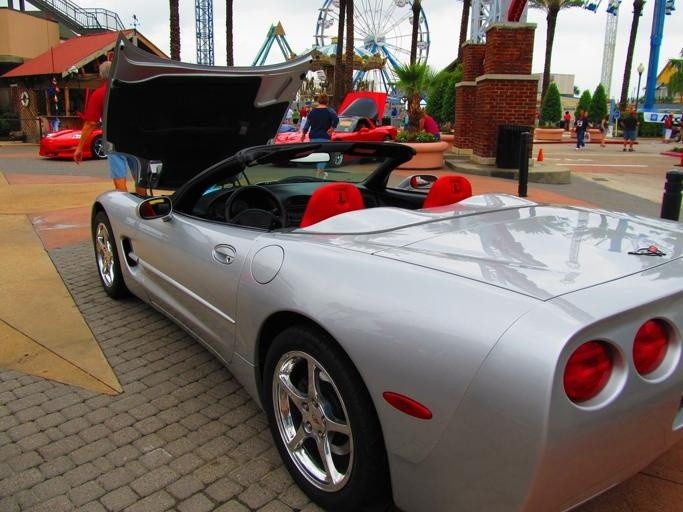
496, 124, 531, 169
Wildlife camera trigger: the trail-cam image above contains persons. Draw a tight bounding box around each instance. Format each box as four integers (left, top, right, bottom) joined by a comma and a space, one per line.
564, 111, 570, 131
416, 111, 439, 141
677, 112, 683, 143
73, 60, 147, 198
285, 106, 309, 131
619, 110, 641, 152
303, 94, 338, 181
390, 106, 397, 119
600, 115, 609, 148
572, 110, 587, 150
664, 112, 673, 142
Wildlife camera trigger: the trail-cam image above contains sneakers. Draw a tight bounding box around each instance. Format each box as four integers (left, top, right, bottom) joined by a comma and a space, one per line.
576, 146, 585, 150
623, 148, 636, 151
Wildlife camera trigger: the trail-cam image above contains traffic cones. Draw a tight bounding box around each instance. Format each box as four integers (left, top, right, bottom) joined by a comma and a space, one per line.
672, 153, 683, 167
536, 147, 545, 162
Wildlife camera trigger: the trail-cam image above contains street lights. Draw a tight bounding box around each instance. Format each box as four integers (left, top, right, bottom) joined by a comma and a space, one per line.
634, 60, 646, 113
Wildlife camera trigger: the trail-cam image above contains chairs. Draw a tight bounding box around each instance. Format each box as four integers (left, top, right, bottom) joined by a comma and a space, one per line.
299, 182, 366, 228
422, 174, 473, 208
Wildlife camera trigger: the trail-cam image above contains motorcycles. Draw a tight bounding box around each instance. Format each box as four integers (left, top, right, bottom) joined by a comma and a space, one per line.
670, 120, 682, 142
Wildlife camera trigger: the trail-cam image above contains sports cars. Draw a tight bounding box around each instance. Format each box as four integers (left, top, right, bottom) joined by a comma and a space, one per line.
37, 124, 108, 163
273, 89, 400, 146
86, 25, 683, 512
